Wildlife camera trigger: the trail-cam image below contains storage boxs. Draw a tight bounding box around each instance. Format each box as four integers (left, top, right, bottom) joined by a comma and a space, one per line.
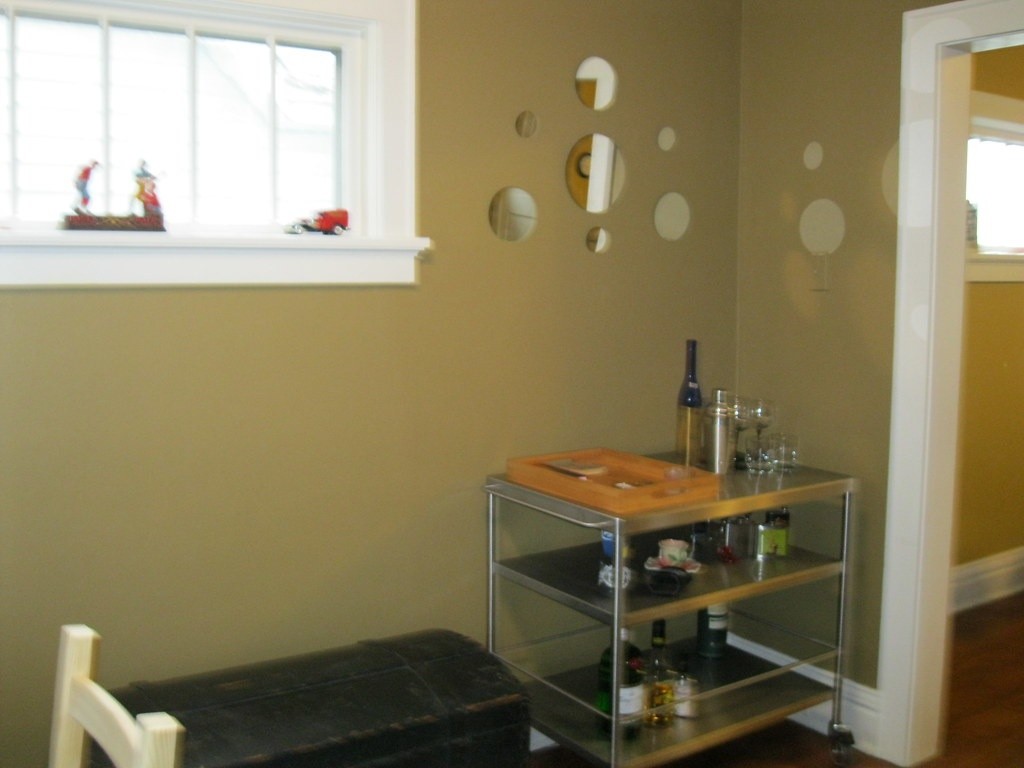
91, 628, 531, 768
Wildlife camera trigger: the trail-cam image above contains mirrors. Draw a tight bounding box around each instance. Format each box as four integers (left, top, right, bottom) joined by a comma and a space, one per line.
575, 55, 619, 110
586, 224, 610, 252
565, 131, 627, 213
488, 187, 536, 242
515, 108, 539, 138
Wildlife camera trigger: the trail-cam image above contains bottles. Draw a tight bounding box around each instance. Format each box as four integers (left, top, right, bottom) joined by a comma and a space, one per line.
677, 339, 703, 463
597, 620, 681, 737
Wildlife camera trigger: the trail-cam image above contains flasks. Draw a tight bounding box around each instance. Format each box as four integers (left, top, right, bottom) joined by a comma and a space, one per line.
703, 388, 735, 475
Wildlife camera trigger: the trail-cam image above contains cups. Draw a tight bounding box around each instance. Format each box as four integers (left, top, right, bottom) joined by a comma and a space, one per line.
745, 433, 797, 476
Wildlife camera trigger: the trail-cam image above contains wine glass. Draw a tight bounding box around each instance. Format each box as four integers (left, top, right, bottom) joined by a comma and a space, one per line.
731, 394, 775, 463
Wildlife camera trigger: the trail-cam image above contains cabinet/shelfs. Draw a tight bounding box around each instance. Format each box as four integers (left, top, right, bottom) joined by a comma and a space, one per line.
483, 448, 862, 768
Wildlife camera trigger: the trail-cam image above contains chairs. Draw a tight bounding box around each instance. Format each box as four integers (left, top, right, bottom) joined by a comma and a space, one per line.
49, 624, 186, 768
